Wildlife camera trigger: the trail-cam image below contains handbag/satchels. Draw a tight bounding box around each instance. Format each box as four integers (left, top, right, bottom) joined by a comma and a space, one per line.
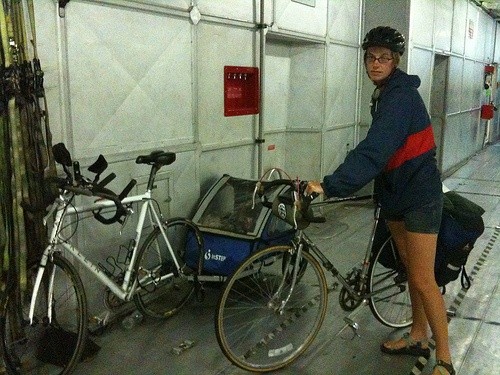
372, 191, 486, 295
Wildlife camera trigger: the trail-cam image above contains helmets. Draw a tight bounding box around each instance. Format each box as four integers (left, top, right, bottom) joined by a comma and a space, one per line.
362, 26, 406, 56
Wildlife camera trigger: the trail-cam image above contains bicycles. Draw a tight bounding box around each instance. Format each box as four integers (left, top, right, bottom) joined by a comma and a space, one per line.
214, 168, 446, 372
0, 143, 206, 375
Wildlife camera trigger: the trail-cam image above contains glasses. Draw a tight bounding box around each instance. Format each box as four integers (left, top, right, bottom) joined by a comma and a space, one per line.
366, 53, 394, 64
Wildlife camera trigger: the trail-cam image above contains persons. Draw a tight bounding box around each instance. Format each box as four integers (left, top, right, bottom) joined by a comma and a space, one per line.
305, 26, 456, 375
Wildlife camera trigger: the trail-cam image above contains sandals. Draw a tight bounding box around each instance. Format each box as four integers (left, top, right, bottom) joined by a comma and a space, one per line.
433, 361, 455, 375
381, 332, 430, 356
